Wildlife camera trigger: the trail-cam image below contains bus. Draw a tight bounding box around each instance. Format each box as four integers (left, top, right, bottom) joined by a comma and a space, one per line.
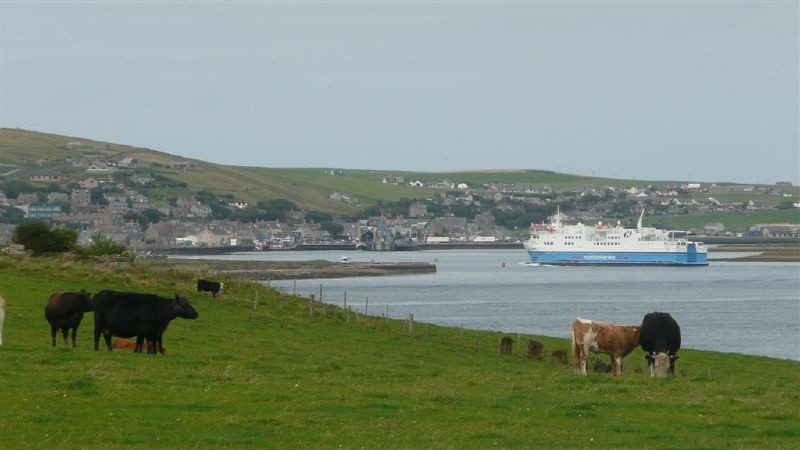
341, 257, 352, 264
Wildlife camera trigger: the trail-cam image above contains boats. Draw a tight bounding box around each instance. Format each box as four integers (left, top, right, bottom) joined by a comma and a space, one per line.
523, 204, 710, 267
256, 238, 298, 250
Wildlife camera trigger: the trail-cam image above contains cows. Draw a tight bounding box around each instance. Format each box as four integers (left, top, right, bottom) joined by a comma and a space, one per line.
569, 312, 682, 379
197, 278, 224, 298
44, 288, 199, 357
501, 337, 513, 352
553, 348, 567, 362
527, 338, 544, 360
